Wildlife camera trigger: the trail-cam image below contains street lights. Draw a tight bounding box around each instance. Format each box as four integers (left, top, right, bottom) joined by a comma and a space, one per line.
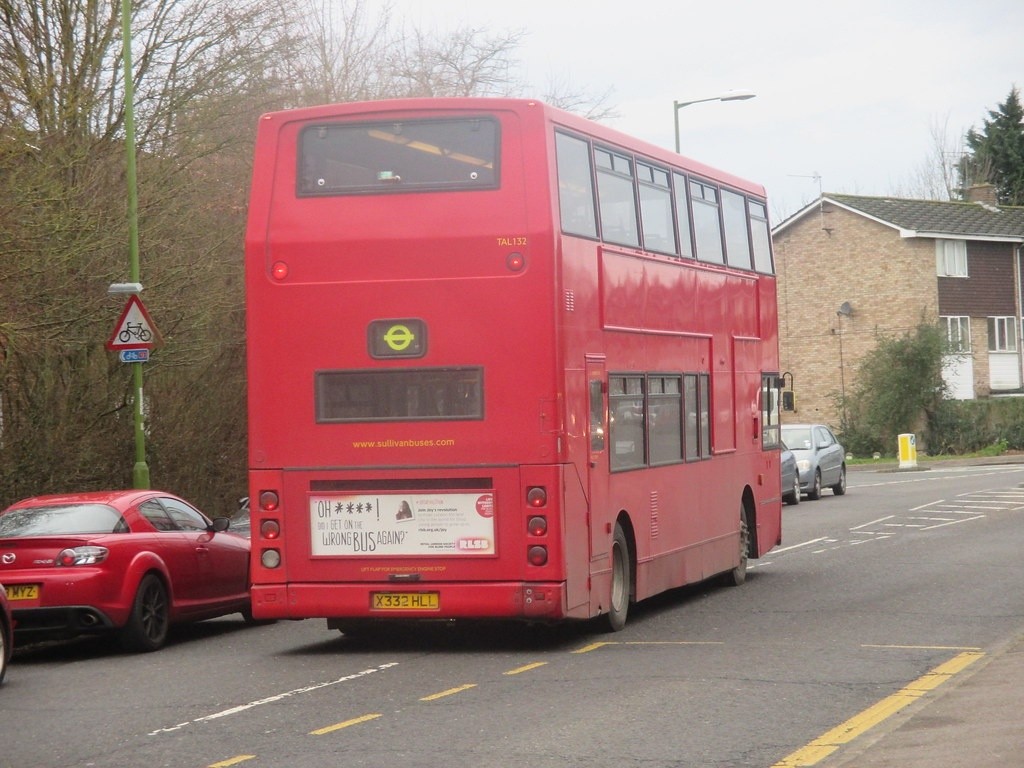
672, 94, 758, 155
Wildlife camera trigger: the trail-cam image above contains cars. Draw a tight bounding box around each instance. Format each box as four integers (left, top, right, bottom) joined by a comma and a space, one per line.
782, 423, 848, 500
0, 488, 278, 653
763, 431, 801, 505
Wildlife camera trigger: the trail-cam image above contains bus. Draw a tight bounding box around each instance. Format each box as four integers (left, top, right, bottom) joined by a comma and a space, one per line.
241, 94, 795, 642
